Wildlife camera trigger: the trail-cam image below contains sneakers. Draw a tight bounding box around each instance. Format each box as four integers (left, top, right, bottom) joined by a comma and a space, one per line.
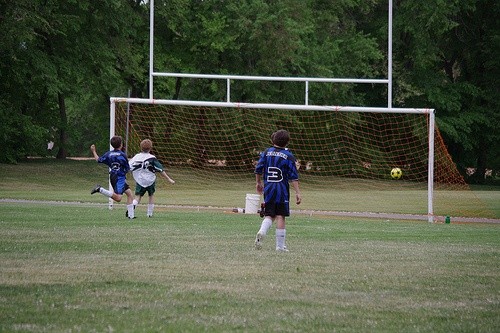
128, 217, 138, 220
91, 183, 103, 195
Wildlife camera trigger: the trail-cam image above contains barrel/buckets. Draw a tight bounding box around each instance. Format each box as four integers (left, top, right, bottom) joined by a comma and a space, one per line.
245, 194, 261, 214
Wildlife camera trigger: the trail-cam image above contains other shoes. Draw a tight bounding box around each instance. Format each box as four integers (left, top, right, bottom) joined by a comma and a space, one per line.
255, 231, 264, 250
126, 210, 128, 217
275, 246, 289, 252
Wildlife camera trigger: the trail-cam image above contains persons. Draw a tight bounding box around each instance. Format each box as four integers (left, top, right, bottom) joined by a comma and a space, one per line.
254, 130, 301, 252
91, 136, 175, 219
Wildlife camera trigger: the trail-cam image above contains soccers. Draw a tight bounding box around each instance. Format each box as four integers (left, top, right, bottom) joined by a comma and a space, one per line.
391, 167, 402, 180
